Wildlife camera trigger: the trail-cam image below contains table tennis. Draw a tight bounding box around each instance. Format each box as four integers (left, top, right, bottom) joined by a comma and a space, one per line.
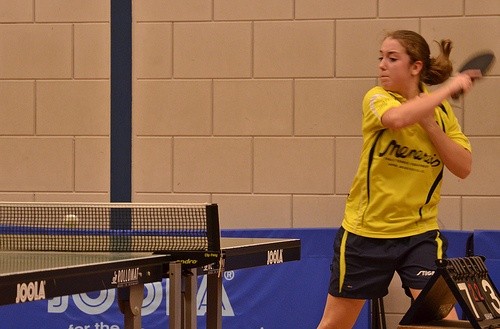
64, 215, 77, 228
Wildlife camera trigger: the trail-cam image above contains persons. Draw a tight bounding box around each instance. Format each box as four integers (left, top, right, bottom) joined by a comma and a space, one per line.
316, 30, 482, 329
